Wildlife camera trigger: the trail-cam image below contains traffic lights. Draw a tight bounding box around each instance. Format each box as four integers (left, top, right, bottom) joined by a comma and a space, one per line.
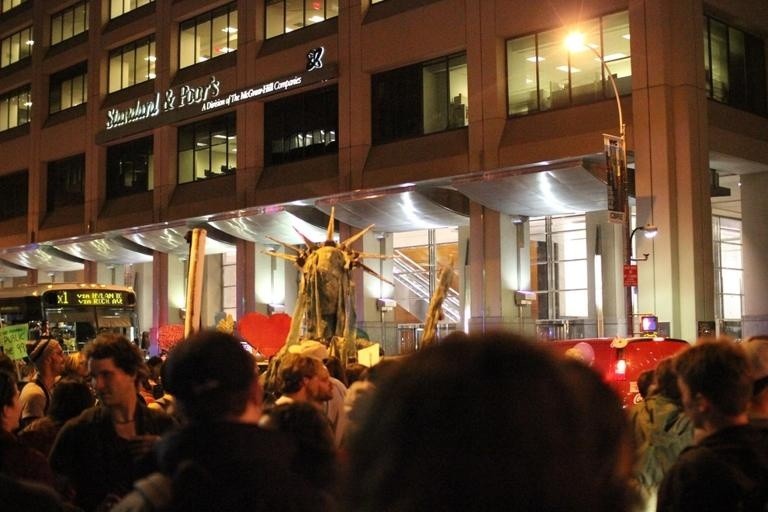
641, 316, 657, 333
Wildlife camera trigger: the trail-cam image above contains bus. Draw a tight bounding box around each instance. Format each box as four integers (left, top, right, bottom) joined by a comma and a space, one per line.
0, 282, 142, 364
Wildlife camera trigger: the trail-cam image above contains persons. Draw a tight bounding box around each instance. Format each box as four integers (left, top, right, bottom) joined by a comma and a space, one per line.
1, 329, 767, 512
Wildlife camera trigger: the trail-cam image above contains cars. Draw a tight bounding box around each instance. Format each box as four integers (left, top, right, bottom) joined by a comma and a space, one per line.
545, 338, 693, 416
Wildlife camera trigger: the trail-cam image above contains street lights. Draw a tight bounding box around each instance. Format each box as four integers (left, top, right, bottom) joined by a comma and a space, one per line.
565, 30, 657, 337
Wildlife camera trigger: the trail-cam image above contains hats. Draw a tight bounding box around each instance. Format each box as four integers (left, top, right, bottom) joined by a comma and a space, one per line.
25, 339, 59, 365
288, 341, 327, 362
